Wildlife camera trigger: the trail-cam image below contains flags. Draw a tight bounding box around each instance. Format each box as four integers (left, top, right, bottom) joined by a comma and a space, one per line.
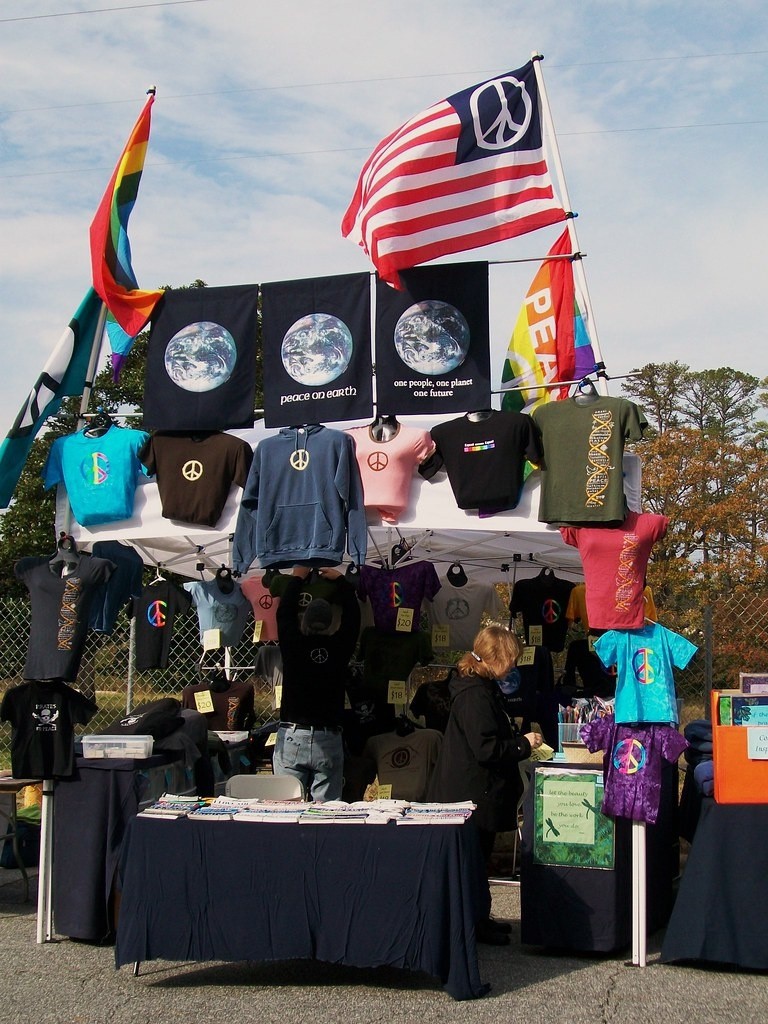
90, 97, 166, 388
0, 284, 103, 511
500, 228, 600, 486
339, 62, 570, 295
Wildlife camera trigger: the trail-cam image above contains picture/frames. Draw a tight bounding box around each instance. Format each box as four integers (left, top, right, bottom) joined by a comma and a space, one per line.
719, 672, 768, 726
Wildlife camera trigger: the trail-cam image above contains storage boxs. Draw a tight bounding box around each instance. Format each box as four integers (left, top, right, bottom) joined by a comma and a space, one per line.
558, 722, 605, 763
80, 735, 155, 759
709, 689, 768, 804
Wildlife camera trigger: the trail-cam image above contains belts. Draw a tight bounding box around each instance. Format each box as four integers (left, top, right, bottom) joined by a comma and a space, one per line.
279, 724, 342, 733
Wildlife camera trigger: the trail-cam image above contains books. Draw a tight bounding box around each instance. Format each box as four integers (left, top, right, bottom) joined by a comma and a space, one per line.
136, 789, 477, 826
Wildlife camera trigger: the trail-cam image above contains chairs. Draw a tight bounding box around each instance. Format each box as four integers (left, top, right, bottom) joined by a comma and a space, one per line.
225, 774, 305, 801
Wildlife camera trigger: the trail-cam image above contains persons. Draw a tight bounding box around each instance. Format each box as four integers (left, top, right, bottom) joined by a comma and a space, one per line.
275, 564, 361, 806
429, 626, 545, 946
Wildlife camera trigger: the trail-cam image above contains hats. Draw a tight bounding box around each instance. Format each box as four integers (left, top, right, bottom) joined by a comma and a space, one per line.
300, 598, 342, 636
447, 564, 468, 587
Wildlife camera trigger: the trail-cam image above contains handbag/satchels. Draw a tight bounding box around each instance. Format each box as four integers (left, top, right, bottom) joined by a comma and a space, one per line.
460, 754, 523, 835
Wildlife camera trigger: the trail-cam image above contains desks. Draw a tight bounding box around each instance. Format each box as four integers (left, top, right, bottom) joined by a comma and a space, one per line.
520, 759, 682, 954
0, 779, 43, 903
54, 740, 222, 942
659, 798, 768, 974
115, 808, 512, 1001
